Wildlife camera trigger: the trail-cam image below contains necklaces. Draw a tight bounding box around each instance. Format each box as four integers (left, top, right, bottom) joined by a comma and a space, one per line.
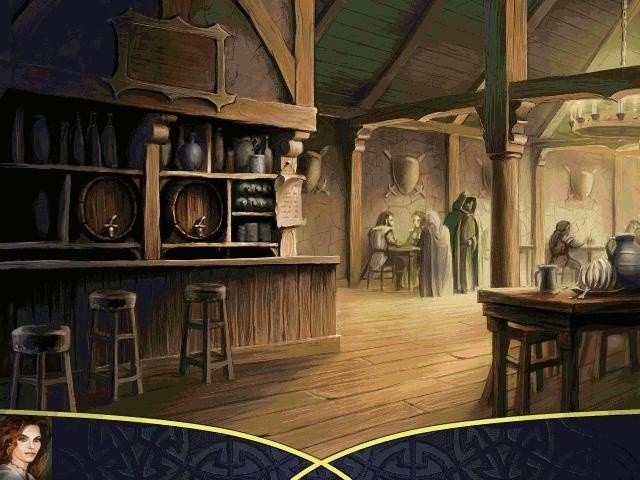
10, 460, 30, 471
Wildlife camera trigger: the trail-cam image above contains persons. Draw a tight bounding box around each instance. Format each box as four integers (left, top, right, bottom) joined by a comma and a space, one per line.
443, 192, 483, 294
400, 210, 425, 289
416, 210, 453, 297
549, 221, 581, 284
0, 413, 53, 480
367, 211, 406, 291
